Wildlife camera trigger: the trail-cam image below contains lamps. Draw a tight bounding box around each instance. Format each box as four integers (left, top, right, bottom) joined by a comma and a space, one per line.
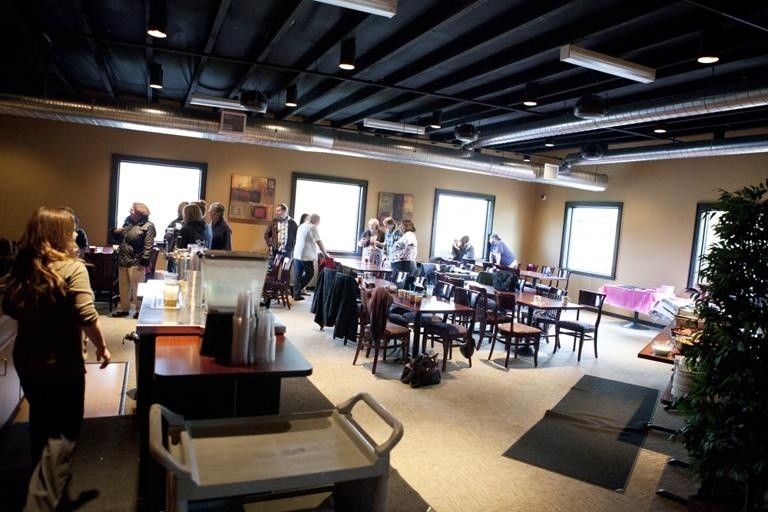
284, 81, 298, 108
148, 63, 163, 90
523, 155, 531, 162
313, 0, 399, 19
429, 110, 442, 129
544, 137, 555, 147
189, 84, 268, 114
654, 121, 668, 133
146, 0, 169, 40
559, 44, 656, 84
522, 81, 538, 107
337, 35, 357, 71
363, 117, 425, 135
695, 42, 720, 65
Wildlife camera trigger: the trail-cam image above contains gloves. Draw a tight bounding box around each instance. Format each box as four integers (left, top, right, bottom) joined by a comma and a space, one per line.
139, 258, 150, 267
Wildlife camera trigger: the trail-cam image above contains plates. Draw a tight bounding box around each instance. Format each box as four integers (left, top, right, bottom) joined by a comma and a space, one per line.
671, 355, 699, 397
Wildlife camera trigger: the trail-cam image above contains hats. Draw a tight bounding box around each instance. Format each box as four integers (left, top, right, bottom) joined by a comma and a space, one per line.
490, 234, 501, 241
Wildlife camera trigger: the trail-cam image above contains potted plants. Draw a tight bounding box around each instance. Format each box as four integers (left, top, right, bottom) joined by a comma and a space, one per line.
672, 178, 768, 512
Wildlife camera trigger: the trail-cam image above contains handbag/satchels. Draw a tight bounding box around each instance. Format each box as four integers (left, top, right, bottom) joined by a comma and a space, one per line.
400, 352, 442, 389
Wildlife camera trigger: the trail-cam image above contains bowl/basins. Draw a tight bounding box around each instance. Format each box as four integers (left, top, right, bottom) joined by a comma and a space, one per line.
670, 323, 703, 353
651, 340, 674, 356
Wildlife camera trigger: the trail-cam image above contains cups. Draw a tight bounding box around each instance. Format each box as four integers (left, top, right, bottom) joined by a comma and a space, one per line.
88, 244, 121, 255
397, 285, 436, 309
562, 296, 568, 307
533, 296, 542, 305
163, 252, 203, 325
231, 284, 275, 367
357, 271, 376, 285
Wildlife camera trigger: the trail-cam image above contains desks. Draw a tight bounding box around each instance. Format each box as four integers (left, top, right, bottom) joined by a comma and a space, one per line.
638, 314, 704, 437
602, 284, 673, 330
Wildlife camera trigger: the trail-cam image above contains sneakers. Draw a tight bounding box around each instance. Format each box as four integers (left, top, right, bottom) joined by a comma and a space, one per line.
290, 285, 311, 301
111, 311, 139, 319
260, 298, 271, 309
56, 488, 99, 512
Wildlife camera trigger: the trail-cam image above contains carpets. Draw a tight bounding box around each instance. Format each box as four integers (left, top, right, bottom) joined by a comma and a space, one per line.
83, 361, 130, 418
502, 374, 661, 495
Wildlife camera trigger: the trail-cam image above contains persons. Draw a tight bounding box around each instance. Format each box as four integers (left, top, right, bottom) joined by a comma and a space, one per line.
112, 200, 232, 319
2, 205, 111, 512
301, 213, 312, 296
259, 204, 297, 306
73, 217, 88, 251
489, 234, 517, 269
452, 236, 470, 260
293, 214, 330, 301
357, 216, 417, 289
